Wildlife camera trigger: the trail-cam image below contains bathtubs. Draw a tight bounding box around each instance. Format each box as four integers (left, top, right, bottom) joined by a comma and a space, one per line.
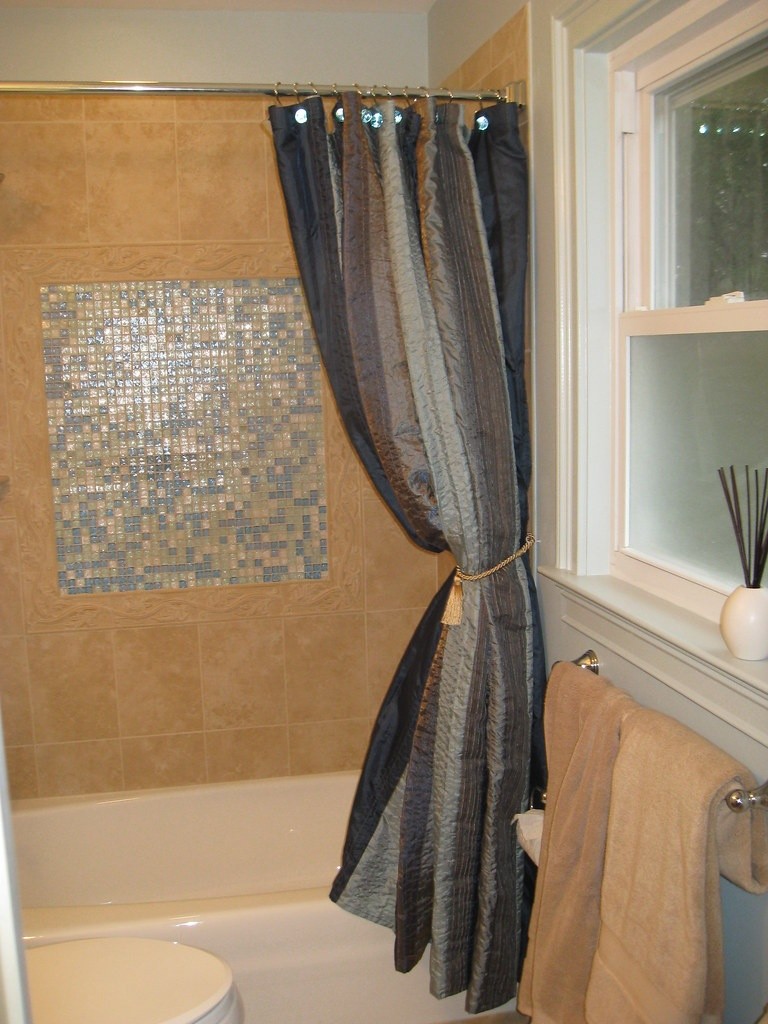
9, 774, 524, 1024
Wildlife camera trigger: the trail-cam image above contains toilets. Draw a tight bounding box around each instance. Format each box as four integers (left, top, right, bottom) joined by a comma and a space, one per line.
0, 938, 243, 1023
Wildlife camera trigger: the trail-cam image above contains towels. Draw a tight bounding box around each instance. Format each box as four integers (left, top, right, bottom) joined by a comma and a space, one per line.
585, 709, 767, 1024
518, 659, 643, 1020
